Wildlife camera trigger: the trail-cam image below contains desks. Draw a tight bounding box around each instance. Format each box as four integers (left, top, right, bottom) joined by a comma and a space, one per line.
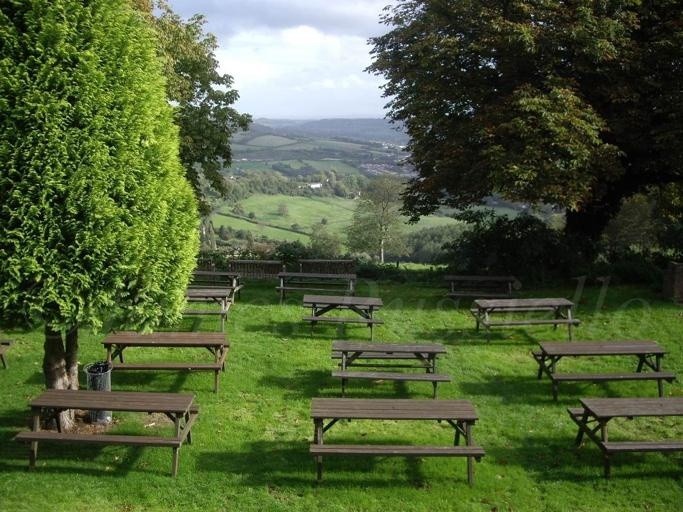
310, 395, 479, 486
28, 386, 192, 485
576, 395, 683, 475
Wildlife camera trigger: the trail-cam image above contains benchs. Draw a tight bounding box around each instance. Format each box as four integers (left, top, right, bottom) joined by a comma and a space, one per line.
566, 406, 683, 472
12, 399, 199, 472
72, 270, 671, 395
308, 443, 488, 491
